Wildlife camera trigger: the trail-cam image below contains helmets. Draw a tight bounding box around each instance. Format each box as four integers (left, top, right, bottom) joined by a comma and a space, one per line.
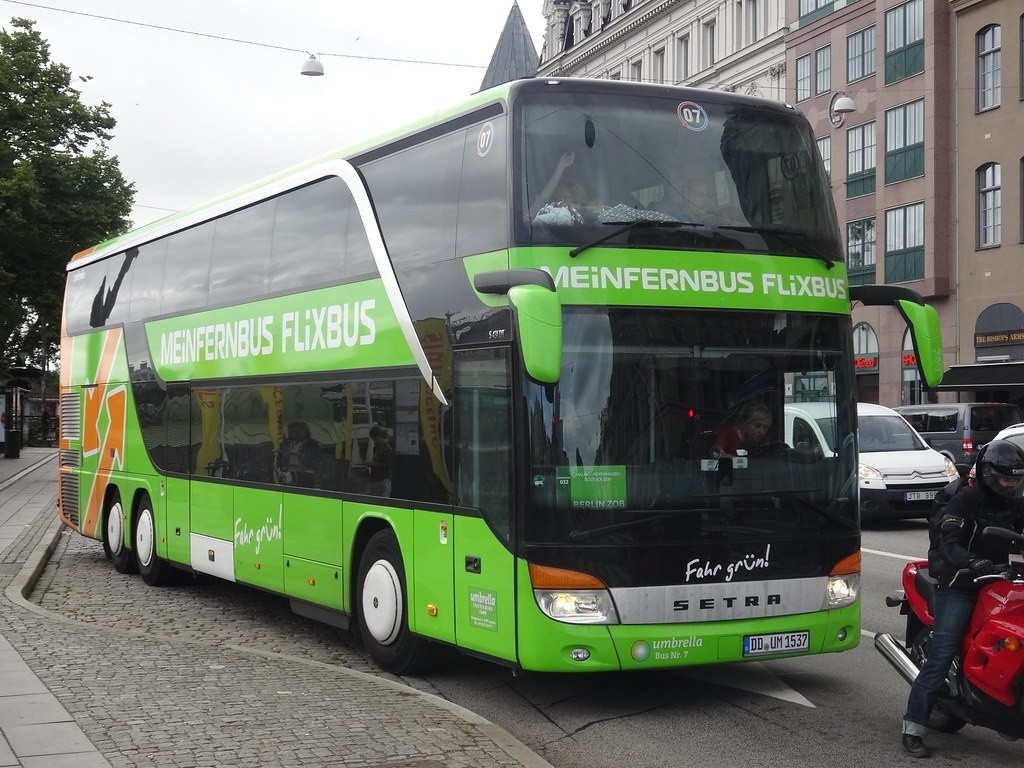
976, 440, 1024, 506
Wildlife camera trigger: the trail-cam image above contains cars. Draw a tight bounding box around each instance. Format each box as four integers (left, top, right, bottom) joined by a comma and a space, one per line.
967, 421, 1024, 489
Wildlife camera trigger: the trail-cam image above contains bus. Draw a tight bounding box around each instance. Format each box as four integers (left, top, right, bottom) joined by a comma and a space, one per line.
55, 75, 946, 679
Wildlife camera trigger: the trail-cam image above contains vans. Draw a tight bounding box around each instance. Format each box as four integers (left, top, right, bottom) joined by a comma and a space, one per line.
886, 400, 1022, 486
780, 398, 960, 525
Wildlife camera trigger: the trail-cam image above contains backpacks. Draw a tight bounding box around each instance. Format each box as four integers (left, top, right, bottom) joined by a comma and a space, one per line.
928, 475, 985, 578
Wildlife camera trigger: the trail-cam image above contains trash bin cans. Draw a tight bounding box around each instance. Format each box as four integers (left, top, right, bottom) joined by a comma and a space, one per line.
4, 430, 21, 458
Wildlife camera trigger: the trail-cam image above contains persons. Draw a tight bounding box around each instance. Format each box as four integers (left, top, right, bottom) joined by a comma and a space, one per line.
140, 404, 152, 428
527, 149, 734, 226
366, 427, 394, 497
903, 439, 1024, 758
41, 406, 50, 441
708, 400, 772, 458
273, 422, 321, 484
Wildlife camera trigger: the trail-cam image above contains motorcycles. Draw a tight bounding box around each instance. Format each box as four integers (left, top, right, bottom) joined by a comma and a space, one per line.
875, 524, 1024, 750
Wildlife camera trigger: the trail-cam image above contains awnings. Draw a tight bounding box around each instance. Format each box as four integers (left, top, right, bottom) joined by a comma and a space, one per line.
922, 362, 1024, 389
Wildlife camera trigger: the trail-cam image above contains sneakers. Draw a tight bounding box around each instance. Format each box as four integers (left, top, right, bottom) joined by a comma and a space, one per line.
902, 734, 930, 757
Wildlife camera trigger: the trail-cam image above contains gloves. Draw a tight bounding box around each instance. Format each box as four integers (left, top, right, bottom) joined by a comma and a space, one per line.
969, 558, 1001, 585
